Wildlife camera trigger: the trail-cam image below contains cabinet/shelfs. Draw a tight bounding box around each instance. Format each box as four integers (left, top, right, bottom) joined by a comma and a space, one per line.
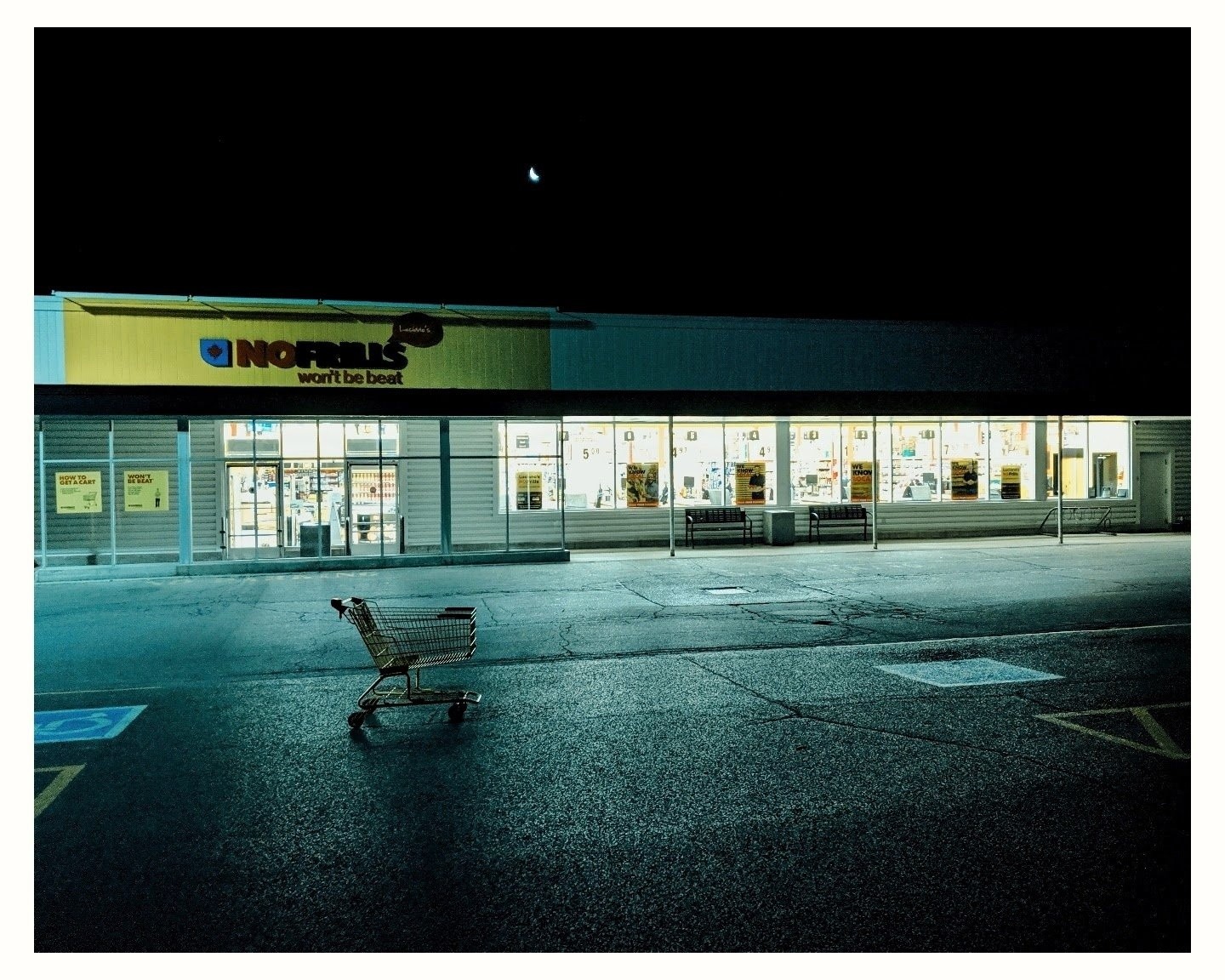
793, 457, 991, 503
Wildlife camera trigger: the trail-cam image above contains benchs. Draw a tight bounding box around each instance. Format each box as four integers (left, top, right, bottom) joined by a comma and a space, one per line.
808, 504, 873, 545
684, 507, 753, 549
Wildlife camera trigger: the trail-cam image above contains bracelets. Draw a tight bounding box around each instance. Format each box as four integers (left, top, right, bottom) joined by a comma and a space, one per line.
651, 496, 653, 499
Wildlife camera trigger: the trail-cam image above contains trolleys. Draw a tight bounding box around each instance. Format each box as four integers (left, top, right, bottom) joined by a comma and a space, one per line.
331, 597, 482, 727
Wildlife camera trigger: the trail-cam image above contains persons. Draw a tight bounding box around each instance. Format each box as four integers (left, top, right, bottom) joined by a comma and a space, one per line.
749, 466, 766, 499
963, 464, 977, 495
641, 473, 658, 503
